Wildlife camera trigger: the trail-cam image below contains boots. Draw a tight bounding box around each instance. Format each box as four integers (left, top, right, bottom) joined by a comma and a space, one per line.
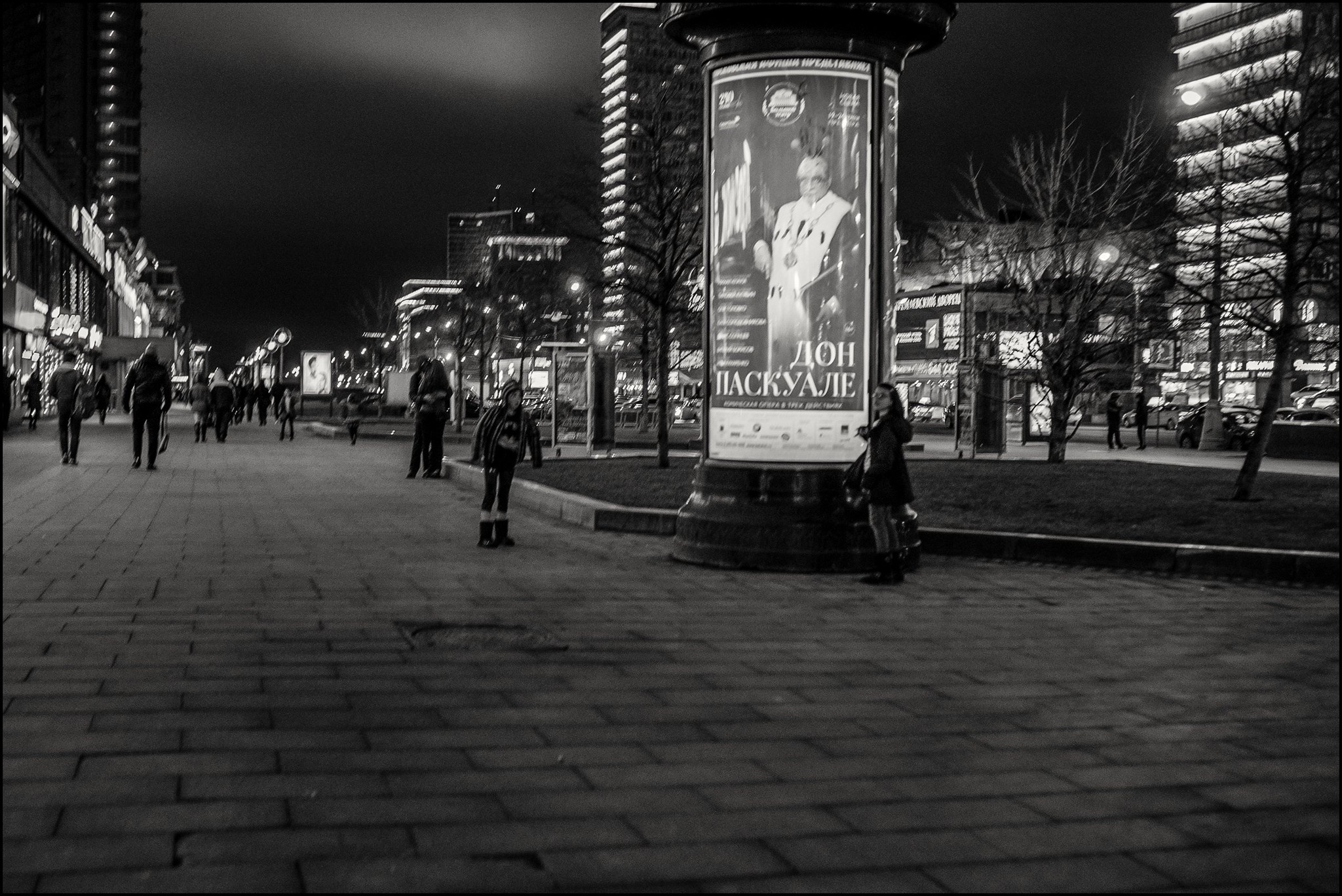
494, 519, 516, 547
477, 522, 496, 549
859, 546, 908, 585
194, 421, 208, 443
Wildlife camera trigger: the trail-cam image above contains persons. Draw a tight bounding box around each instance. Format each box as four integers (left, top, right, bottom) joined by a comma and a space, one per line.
477, 378, 543, 549
0, 364, 17, 430
746, 154, 863, 395
123, 341, 173, 471
1134, 393, 1148, 450
174, 358, 364, 446
407, 358, 454, 480
23, 371, 42, 430
48, 352, 85, 464
305, 356, 326, 394
93, 372, 111, 425
1105, 392, 1128, 449
861, 382, 922, 586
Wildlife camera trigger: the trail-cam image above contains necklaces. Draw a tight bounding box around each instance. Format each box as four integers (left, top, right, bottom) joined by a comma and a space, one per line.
784, 200, 835, 270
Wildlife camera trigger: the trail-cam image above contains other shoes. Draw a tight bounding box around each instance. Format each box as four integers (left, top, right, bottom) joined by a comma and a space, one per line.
146, 463, 159, 470
406, 464, 441, 479
99, 418, 104, 425
351, 439, 356, 445
279, 434, 293, 441
216, 433, 228, 442
132, 457, 141, 469
60, 452, 79, 465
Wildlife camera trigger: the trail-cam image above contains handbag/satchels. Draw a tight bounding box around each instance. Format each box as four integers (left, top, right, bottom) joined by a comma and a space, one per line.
840, 450, 871, 514
158, 410, 170, 455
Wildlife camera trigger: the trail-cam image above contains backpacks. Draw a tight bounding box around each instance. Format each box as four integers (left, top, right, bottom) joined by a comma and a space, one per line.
73, 370, 98, 420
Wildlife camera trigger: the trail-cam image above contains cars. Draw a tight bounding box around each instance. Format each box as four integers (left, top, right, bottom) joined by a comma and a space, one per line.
905, 402, 932, 420
1123, 406, 1149, 427
1174, 382, 1340, 460
1006, 393, 1083, 425
1149, 404, 1194, 430
944, 401, 956, 428
521, 395, 700, 431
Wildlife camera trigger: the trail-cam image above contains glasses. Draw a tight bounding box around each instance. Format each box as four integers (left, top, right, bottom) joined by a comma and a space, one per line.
871, 392, 888, 400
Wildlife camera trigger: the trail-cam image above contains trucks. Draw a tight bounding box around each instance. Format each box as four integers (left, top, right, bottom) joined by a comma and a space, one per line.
384, 369, 489, 419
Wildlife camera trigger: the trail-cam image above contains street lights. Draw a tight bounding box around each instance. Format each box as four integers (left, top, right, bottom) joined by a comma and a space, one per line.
233, 328, 292, 384
572, 283, 594, 318
1100, 250, 1140, 403
